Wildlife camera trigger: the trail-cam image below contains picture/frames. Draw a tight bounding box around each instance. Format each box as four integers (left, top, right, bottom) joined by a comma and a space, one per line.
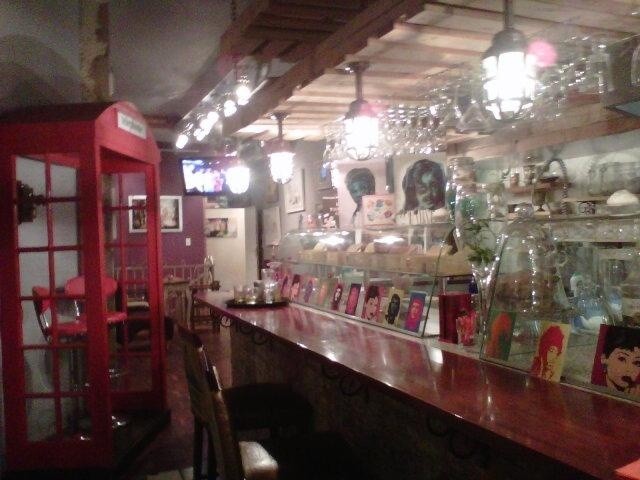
282, 167, 305, 215
127, 195, 185, 235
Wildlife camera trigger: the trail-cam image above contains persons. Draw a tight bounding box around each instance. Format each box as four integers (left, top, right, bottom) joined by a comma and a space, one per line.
279, 273, 424, 331
395, 158, 444, 219
183, 164, 223, 193
346, 167, 376, 222
599, 325, 640, 395
530, 325, 565, 385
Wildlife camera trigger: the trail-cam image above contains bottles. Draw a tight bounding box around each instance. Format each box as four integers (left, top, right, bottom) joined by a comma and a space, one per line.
570, 244, 623, 331
439, 152, 508, 216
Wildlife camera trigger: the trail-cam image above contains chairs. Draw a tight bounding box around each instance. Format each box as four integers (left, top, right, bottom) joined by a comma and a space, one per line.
178, 327, 357, 480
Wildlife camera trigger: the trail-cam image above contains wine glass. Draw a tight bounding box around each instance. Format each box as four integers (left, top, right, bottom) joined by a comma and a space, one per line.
321, 103, 449, 160
454, 185, 509, 348
420, 29, 612, 135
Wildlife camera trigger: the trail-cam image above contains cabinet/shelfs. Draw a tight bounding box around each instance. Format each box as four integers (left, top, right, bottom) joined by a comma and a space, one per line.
278, 222, 472, 344
470, 209, 638, 404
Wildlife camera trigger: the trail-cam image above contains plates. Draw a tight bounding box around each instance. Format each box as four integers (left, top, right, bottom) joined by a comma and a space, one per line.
606, 211, 640, 218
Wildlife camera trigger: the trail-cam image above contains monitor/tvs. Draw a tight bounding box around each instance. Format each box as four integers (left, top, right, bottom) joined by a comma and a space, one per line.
179, 156, 241, 195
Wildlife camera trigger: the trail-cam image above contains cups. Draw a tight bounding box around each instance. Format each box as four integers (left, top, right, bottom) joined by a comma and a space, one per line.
629, 7, 640, 84
233, 280, 280, 304
438, 292, 472, 345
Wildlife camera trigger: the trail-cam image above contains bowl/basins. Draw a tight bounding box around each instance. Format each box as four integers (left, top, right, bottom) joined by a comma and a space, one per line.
607, 203, 638, 213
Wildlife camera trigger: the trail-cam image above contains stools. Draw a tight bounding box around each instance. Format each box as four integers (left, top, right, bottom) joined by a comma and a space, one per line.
31, 273, 128, 442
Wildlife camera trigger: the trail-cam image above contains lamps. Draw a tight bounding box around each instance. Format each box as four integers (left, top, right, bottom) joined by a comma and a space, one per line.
173, 134, 190, 149
223, 76, 252, 116
339, 62, 381, 161
223, 137, 252, 194
266, 111, 296, 184
480, 1, 539, 123
192, 111, 219, 140
